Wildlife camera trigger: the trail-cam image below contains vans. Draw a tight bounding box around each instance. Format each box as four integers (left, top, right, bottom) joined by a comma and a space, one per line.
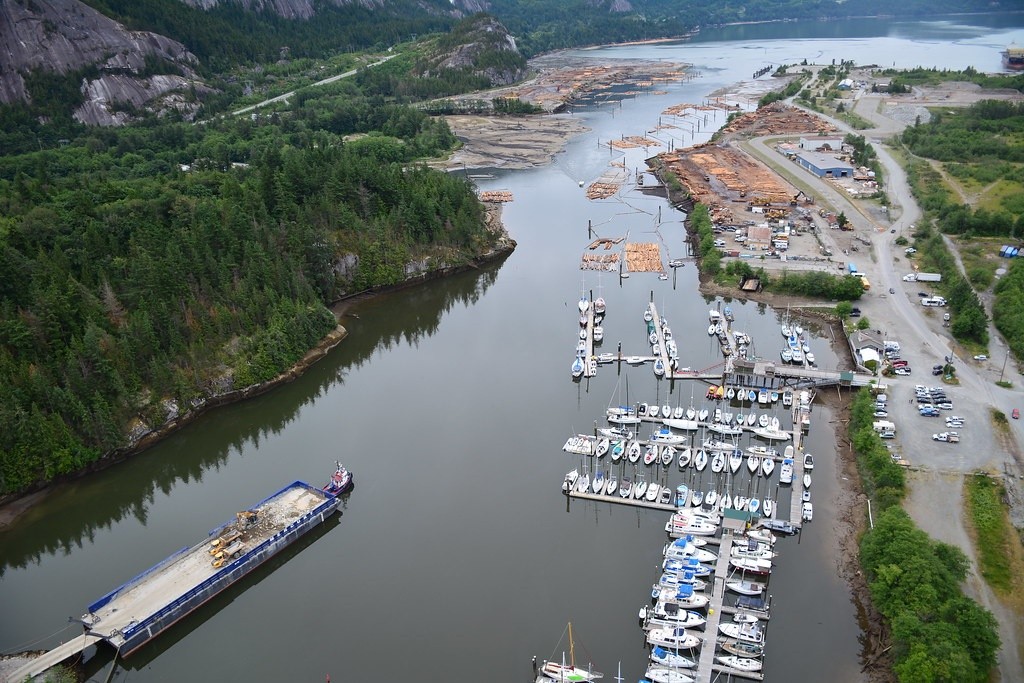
933, 296, 948, 303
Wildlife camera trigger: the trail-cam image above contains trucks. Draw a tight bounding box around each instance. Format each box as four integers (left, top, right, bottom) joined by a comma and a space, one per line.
922, 297, 945, 307
998, 245, 1024, 258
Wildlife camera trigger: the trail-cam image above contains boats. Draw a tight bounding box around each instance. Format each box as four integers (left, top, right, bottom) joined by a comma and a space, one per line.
572, 298, 751, 379
321, 459, 354, 497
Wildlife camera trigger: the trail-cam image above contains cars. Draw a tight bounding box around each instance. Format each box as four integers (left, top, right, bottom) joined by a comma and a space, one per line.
874, 394, 901, 460
885, 348, 987, 376
850, 307, 862, 317
918, 292, 928, 297
711, 218, 816, 257
1012, 408, 1019, 419
915, 385, 965, 444
890, 288, 895, 294
905, 247, 916, 253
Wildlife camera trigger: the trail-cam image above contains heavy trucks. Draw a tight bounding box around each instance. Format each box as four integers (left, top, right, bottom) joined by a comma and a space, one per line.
903, 272, 942, 282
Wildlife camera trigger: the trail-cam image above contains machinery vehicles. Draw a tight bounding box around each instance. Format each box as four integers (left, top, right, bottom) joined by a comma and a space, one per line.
790, 191, 814, 205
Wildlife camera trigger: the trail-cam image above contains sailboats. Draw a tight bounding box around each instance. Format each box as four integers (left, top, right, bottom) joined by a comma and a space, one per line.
529, 385, 815, 683
781, 302, 815, 364
